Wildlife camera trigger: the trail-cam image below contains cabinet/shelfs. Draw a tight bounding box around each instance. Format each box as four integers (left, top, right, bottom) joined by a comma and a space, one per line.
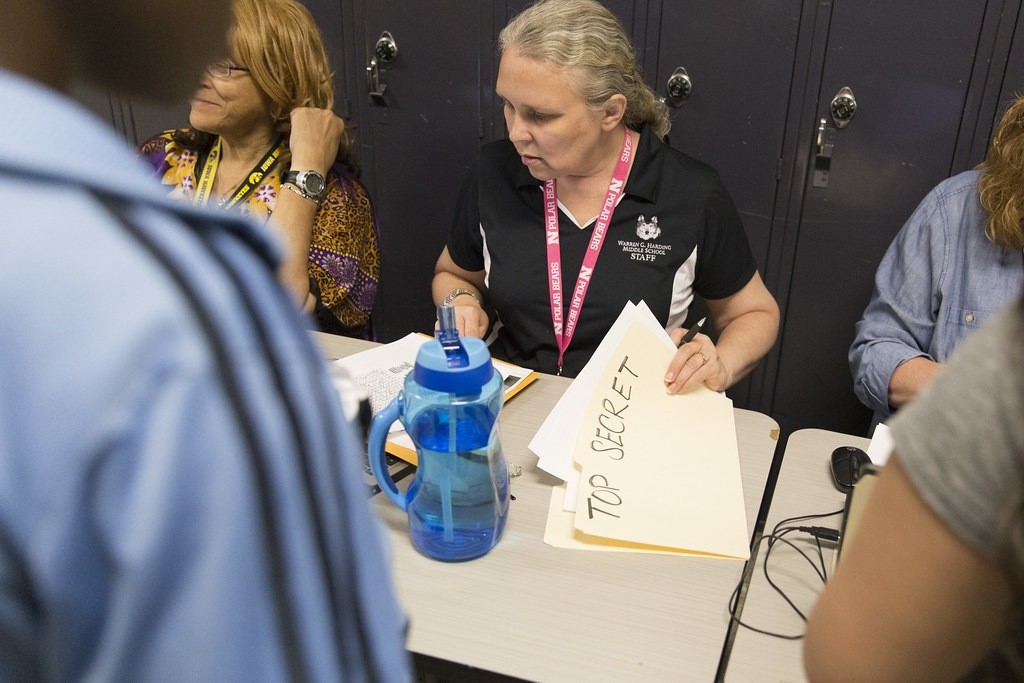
81, 0, 1024, 433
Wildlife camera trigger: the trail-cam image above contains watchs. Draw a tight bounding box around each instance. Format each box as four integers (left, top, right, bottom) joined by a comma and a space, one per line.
280, 169, 327, 199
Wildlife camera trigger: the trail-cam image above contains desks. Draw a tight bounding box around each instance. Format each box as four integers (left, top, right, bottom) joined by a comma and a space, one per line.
719, 426, 874, 683
305, 329, 783, 683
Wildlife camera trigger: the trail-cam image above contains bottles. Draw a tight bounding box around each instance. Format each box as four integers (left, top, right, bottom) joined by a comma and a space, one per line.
366, 299, 512, 564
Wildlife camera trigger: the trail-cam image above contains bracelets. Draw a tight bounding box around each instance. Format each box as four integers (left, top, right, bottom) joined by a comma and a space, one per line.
279, 184, 320, 205
436, 288, 480, 319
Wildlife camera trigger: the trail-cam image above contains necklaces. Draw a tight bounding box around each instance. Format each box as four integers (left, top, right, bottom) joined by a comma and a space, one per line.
216, 166, 252, 206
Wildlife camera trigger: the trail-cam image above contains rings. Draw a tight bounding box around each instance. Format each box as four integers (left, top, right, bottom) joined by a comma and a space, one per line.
696, 352, 708, 364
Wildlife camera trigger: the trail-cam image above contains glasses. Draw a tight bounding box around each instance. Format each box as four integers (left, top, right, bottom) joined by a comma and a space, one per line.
206, 58, 250, 77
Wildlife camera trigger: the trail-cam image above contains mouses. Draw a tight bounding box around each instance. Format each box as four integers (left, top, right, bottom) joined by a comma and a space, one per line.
831, 447, 873, 493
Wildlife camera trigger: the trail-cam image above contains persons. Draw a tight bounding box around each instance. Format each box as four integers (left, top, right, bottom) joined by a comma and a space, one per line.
124, 2, 381, 345
0, 2, 415, 683
431, 0, 780, 398
802, 92, 1024, 683
